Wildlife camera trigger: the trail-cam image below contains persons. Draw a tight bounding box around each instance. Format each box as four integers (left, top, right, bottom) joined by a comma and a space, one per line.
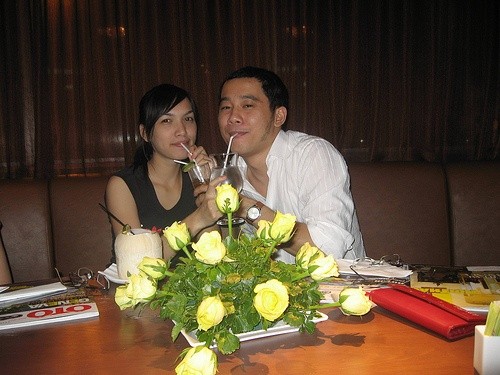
193, 66, 367, 264
0, 238, 12, 284
105, 85, 225, 270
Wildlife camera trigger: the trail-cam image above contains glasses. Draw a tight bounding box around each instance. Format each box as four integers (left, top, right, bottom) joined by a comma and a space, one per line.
52, 266, 111, 292
349, 252, 427, 281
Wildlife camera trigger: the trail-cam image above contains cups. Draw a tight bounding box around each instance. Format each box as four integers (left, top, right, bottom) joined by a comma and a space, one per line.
473, 325, 500, 375
188, 161, 211, 189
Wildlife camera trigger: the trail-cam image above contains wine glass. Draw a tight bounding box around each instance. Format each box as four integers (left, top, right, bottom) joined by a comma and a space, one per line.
209, 152, 245, 226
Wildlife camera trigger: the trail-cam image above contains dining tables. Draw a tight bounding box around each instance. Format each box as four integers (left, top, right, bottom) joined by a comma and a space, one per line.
0, 261, 500, 375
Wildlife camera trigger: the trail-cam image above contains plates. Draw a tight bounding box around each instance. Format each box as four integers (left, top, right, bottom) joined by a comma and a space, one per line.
171, 310, 328, 347
97, 263, 130, 284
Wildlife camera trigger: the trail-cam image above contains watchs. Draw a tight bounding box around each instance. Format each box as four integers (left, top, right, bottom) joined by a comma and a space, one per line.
246, 201, 265, 224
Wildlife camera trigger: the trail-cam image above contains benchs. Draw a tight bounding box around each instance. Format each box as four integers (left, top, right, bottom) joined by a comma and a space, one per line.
0, 161, 500, 284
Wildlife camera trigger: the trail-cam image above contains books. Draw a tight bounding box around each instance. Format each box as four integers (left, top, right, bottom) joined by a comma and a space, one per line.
318, 263, 500, 311
0, 277, 99, 329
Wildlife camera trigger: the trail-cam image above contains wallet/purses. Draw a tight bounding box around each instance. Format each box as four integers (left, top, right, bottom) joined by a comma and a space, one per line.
367, 284, 485, 341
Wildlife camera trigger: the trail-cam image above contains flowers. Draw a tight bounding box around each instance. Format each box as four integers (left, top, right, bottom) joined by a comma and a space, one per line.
115, 186, 376, 375
